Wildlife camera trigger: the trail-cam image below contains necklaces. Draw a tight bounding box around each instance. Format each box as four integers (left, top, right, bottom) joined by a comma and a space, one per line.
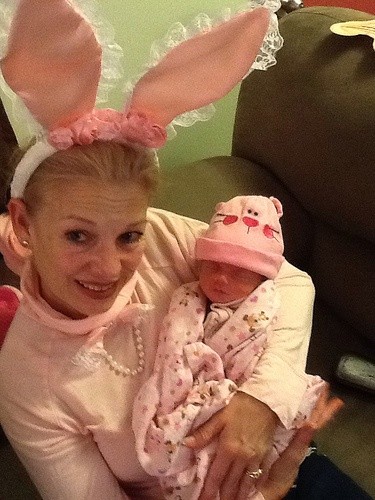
96, 323, 144, 375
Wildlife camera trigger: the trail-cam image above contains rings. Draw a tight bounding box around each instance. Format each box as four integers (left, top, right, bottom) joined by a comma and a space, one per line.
245, 470, 263, 479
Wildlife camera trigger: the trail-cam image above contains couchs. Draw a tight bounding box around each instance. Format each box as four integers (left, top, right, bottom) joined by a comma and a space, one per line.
0, 5, 375, 500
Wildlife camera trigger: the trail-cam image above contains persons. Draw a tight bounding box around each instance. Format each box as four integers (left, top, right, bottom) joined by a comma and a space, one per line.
0, 0, 374, 500
131, 195, 285, 500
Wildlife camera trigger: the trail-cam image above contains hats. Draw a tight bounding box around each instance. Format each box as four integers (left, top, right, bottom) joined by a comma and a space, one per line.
195, 194, 285, 281
